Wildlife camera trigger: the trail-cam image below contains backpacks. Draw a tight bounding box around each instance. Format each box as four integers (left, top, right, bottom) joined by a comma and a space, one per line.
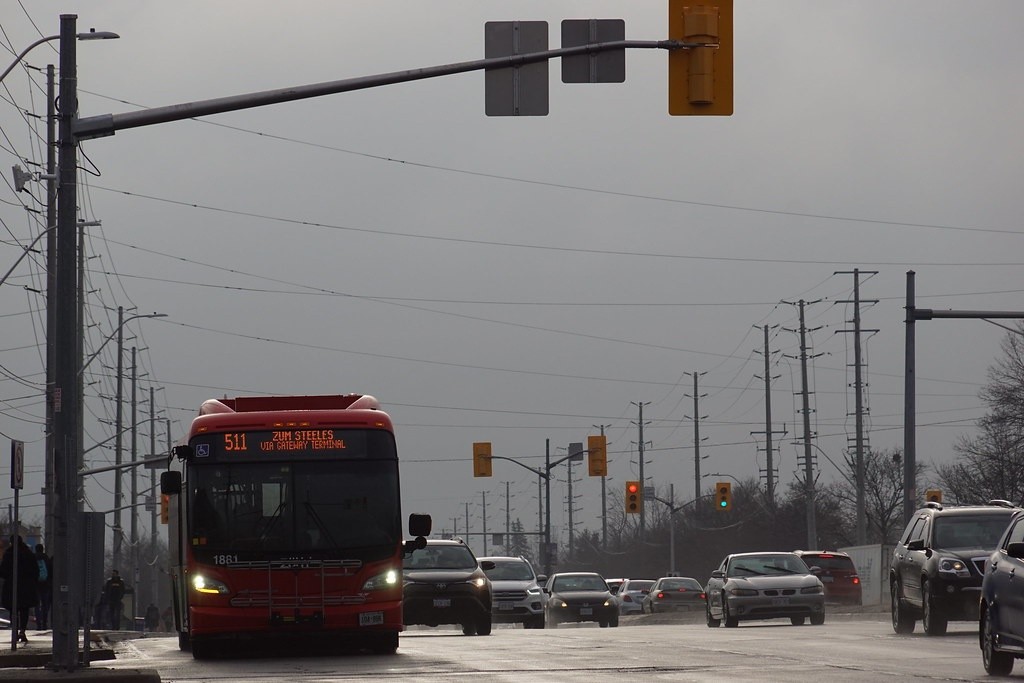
34, 552, 48, 582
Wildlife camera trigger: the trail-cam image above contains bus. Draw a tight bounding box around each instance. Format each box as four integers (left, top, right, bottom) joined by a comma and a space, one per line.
160, 394, 433, 660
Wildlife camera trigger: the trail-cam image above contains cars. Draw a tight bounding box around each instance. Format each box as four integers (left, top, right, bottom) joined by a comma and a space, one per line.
614, 580, 657, 614
979, 510, 1024, 676
797, 550, 862, 606
705, 552, 825, 628
641, 576, 706, 614
545, 573, 620, 628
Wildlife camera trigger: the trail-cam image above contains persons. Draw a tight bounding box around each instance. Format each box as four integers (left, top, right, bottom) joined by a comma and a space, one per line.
33, 543, 51, 630
162, 607, 173, 632
145, 602, 160, 632
105, 570, 124, 630
94, 586, 106, 630
0, 534, 40, 643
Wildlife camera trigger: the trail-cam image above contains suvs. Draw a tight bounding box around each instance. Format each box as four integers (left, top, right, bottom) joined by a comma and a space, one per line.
476, 556, 547, 629
889, 500, 1023, 635
401, 538, 496, 635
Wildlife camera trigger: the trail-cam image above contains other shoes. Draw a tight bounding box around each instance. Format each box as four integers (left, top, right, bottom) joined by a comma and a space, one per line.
17, 629, 28, 643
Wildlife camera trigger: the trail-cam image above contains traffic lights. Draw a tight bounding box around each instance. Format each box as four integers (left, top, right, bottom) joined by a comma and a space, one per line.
716, 483, 731, 511
625, 481, 641, 513
161, 492, 169, 524
927, 491, 942, 503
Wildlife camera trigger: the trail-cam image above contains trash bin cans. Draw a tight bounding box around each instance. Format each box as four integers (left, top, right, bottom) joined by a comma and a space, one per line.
134, 616, 145, 631
